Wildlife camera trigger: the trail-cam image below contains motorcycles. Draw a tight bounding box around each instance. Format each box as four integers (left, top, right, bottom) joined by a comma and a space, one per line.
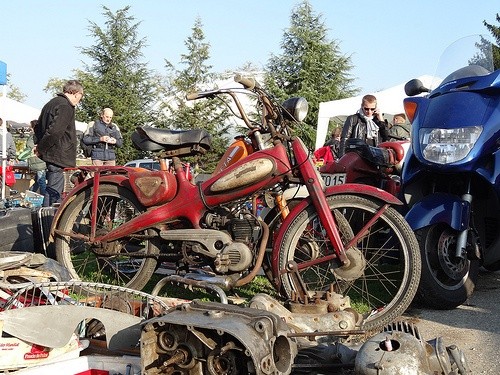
43, 75, 421, 334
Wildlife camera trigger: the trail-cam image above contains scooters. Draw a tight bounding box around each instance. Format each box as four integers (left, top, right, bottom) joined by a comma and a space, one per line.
400, 35, 500, 310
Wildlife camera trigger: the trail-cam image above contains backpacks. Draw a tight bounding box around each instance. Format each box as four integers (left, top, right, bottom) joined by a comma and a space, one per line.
79, 121, 95, 156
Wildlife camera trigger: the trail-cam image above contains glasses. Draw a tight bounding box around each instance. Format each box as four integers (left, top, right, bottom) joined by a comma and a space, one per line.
364, 107, 376, 111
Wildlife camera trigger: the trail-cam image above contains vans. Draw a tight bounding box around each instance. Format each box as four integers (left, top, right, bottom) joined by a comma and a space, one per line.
123, 159, 191, 178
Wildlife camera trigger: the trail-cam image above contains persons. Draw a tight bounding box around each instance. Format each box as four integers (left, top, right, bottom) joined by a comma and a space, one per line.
0, 118, 48, 196
323, 113, 410, 160
339, 94, 387, 161
34, 79, 84, 208
83, 107, 123, 166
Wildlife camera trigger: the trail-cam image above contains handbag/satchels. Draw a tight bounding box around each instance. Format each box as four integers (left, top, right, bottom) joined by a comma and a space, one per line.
0, 165, 16, 187
